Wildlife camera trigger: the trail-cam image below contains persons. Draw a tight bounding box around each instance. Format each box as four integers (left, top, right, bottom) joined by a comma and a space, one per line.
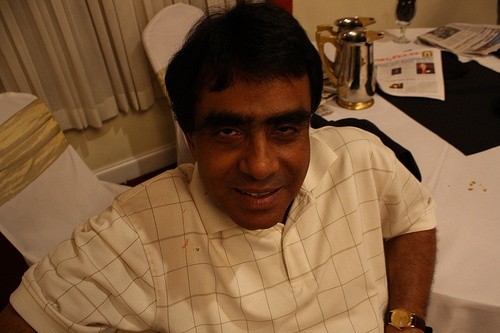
0, 2, 437, 333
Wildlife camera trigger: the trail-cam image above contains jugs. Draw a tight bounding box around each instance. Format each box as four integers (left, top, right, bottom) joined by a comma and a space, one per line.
316, 16, 376, 77
316, 30, 384, 110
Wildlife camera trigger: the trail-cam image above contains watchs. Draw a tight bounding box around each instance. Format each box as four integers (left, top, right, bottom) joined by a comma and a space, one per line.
384, 308, 433, 333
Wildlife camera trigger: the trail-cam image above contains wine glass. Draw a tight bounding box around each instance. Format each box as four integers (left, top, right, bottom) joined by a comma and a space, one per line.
392, 0, 416, 44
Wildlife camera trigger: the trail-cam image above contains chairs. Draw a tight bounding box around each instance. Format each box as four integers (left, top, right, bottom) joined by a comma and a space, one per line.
0, 91, 136, 265
141, 3, 206, 167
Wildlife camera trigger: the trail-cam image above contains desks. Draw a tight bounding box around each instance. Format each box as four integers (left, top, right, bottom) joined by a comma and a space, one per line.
308, 27, 500, 333
